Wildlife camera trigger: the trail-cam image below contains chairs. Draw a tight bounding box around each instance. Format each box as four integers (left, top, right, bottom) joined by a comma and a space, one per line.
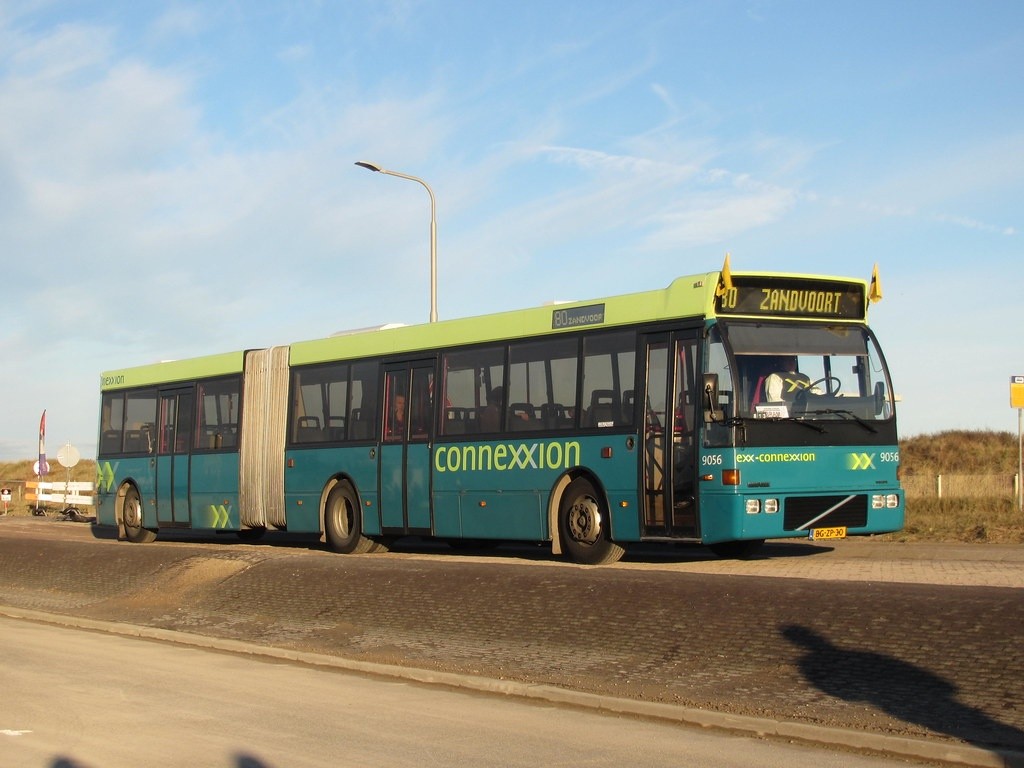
103, 408, 375, 452
445, 382, 731, 434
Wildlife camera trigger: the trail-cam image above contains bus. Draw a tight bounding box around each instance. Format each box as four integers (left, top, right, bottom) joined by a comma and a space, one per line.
92, 259, 910, 565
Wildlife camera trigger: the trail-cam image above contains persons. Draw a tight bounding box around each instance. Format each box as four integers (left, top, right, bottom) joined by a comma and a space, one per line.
480, 386, 528, 432
390, 395, 405, 434
764, 356, 835, 402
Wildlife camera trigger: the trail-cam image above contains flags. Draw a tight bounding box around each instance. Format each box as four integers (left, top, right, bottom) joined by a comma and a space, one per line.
869, 265, 883, 305
715, 255, 733, 298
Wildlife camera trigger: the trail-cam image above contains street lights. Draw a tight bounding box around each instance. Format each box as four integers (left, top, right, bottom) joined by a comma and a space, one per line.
353, 156, 437, 327
